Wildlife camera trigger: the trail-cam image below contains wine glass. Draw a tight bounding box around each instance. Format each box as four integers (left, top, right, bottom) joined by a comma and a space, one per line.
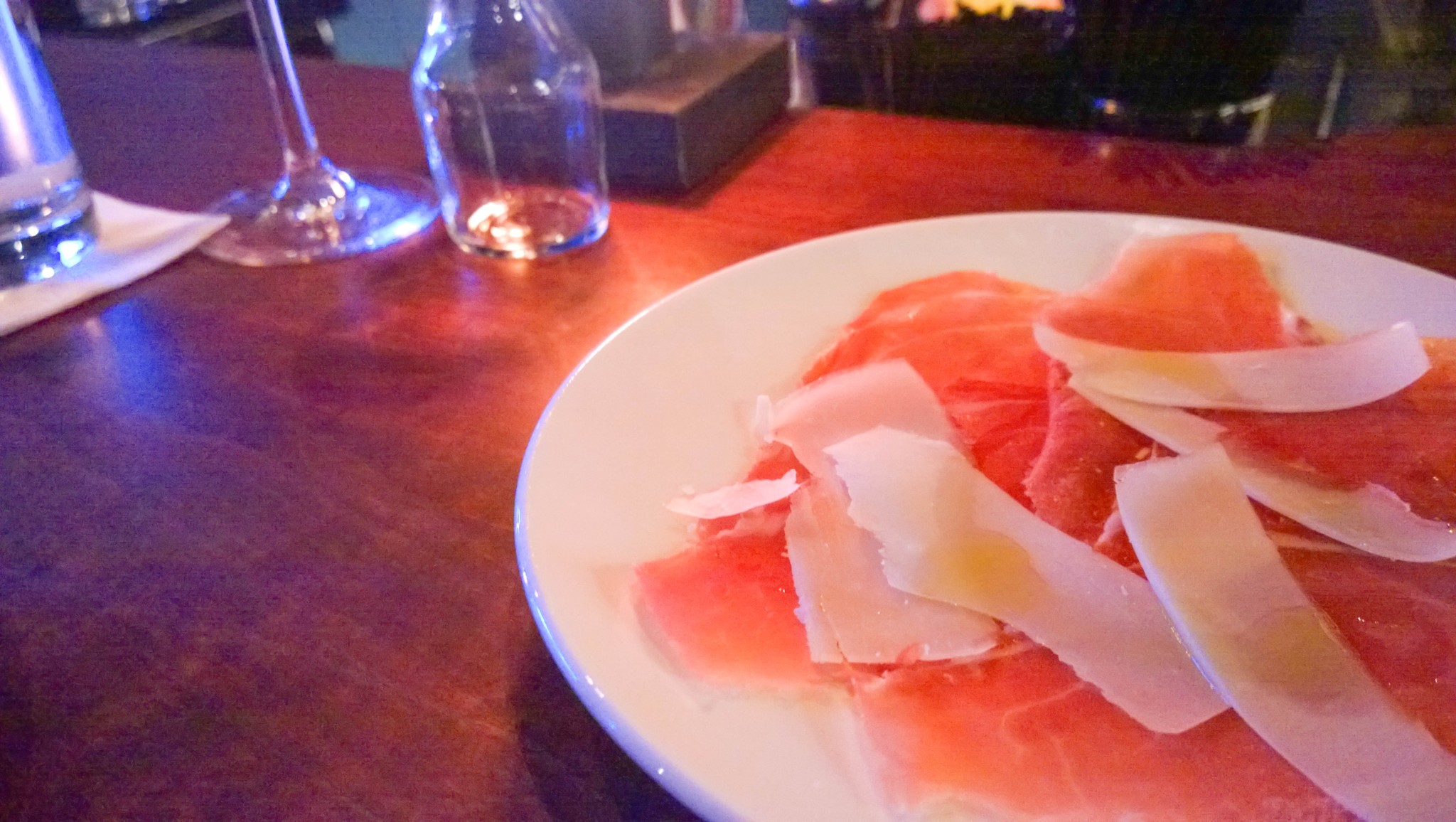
195, 0, 442, 268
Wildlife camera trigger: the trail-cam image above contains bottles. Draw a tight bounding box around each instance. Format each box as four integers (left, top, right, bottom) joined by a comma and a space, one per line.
408, 0, 610, 255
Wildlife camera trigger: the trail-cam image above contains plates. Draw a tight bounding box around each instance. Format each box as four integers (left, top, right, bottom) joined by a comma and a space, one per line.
514, 210, 1454, 821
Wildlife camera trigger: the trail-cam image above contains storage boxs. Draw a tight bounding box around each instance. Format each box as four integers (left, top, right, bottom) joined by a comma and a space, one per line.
586, 20, 792, 196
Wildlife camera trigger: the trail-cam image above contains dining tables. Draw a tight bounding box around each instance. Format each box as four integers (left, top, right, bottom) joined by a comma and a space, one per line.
1, 30, 1456, 820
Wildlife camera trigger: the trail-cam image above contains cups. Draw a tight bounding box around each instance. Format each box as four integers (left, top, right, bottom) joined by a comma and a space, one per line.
0, 0, 99, 289
